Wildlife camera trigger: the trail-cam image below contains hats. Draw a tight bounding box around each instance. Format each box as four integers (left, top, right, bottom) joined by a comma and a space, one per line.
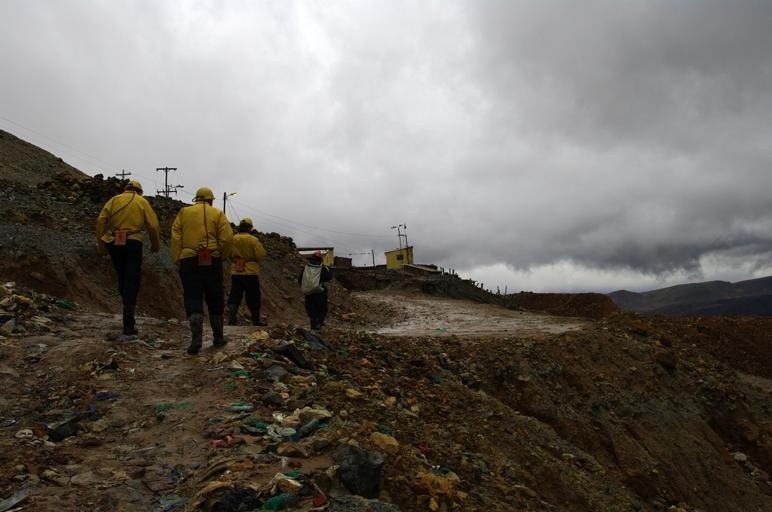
310, 252, 323, 265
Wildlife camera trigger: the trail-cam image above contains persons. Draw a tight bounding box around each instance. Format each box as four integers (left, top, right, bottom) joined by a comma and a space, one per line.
170, 187, 233, 353
96, 180, 160, 335
298, 253, 332, 330
227, 217, 267, 326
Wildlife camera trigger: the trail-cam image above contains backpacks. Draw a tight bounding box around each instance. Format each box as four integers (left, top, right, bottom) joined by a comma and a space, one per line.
301, 264, 325, 295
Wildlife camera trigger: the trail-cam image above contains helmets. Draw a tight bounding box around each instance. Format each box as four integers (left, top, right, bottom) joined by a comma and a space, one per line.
195, 187, 215, 202
239, 217, 253, 228
124, 180, 143, 195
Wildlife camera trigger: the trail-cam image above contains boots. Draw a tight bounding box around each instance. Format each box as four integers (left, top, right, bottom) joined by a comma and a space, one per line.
227, 300, 239, 326
187, 313, 204, 355
209, 313, 229, 347
251, 309, 267, 326
124, 305, 138, 335
